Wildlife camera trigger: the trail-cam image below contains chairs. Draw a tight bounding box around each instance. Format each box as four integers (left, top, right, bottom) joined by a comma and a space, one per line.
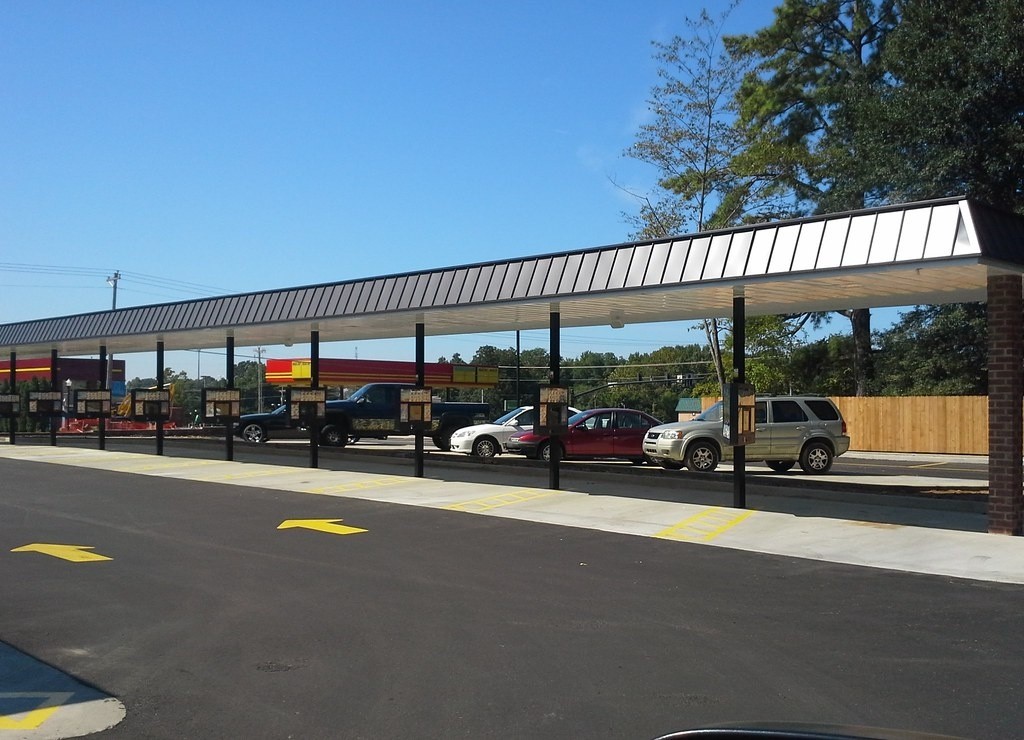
595, 416, 602, 429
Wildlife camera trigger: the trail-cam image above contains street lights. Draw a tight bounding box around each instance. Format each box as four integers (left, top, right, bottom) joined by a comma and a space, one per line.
106, 277, 118, 391
66, 378, 72, 431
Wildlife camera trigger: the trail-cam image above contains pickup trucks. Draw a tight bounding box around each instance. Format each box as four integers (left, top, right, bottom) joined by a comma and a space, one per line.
296, 383, 490, 450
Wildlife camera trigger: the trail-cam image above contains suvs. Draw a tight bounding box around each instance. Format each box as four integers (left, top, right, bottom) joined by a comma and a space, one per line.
233, 404, 357, 444
642, 394, 850, 475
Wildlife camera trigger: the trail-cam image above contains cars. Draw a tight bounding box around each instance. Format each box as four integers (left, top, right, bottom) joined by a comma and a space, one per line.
449, 405, 597, 458
503, 408, 664, 462
